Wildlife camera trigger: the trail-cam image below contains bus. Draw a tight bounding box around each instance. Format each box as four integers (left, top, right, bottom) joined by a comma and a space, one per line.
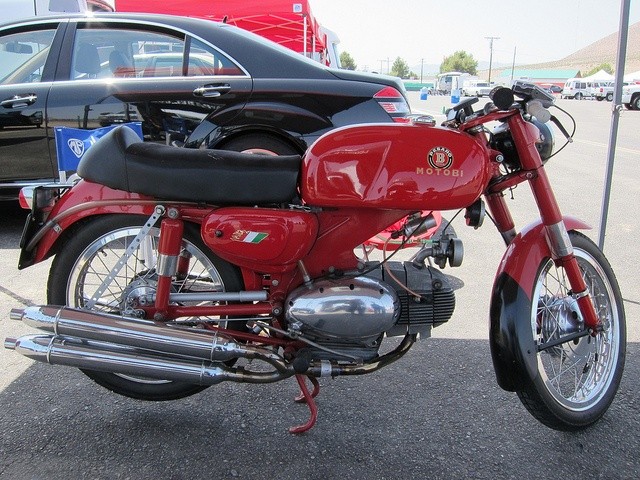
563, 78, 612, 101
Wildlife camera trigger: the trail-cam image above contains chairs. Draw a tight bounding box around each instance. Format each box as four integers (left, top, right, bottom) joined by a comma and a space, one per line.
76, 44, 100, 81
110, 50, 134, 78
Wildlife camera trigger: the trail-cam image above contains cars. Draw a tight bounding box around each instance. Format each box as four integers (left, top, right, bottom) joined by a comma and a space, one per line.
621, 84, 640, 110
0, 13, 413, 215
550, 85, 564, 93
73, 52, 222, 79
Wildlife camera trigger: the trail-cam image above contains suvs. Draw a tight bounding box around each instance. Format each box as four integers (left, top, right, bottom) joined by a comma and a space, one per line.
462, 79, 493, 97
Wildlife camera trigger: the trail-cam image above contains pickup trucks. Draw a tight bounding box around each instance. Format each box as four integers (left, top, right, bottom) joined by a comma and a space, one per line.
590, 82, 638, 101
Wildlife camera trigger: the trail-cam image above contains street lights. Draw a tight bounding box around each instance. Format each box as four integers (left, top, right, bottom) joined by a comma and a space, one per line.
485, 37, 500, 86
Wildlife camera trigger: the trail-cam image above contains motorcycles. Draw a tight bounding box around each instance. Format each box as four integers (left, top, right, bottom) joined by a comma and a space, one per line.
4, 76, 627, 432
